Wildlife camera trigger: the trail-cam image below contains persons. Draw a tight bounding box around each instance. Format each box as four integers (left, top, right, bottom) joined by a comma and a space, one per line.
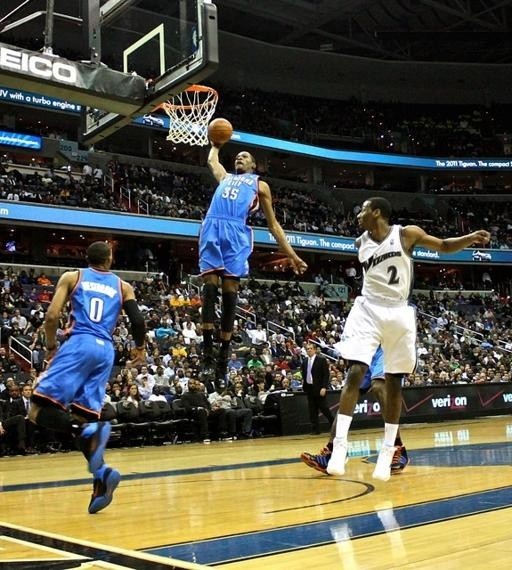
1, 94, 512, 458
302, 198, 490, 482
33, 241, 146, 514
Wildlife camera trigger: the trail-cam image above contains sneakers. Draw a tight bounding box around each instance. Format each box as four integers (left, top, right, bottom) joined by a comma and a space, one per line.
203, 350, 218, 375
214, 368, 230, 390
373, 443, 410, 483
300, 439, 348, 476
11, 431, 254, 456
80, 421, 113, 473
88, 464, 121, 513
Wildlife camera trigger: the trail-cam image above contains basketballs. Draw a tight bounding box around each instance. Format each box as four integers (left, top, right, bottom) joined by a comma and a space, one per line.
208, 118, 233, 143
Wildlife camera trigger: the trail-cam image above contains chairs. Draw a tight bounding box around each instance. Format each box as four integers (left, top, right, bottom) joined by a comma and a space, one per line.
1, 132, 512, 460
